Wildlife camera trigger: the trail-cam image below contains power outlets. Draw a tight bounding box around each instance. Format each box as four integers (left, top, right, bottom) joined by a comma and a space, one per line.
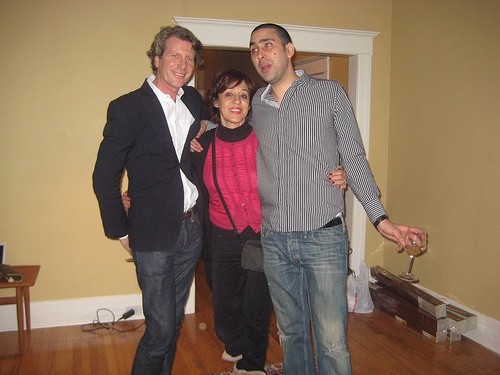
125, 306, 142, 317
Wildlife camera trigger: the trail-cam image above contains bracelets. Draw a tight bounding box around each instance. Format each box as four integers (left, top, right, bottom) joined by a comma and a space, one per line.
373, 215, 388, 228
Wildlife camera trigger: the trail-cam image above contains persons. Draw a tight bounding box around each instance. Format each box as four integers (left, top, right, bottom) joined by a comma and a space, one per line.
121, 70, 348, 374
190, 23, 426, 375
92, 25, 221, 375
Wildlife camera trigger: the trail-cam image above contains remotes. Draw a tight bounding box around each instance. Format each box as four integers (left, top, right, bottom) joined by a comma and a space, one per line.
0, 264, 22, 281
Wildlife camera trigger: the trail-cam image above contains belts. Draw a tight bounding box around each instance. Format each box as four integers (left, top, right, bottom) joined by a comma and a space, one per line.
182, 205, 198, 221
319, 216, 346, 229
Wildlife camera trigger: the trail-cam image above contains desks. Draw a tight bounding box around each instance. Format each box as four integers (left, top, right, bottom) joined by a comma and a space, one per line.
0, 265, 40, 352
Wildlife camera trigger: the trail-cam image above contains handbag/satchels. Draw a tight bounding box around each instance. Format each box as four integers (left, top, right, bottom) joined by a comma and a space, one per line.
241, 239, 265, 273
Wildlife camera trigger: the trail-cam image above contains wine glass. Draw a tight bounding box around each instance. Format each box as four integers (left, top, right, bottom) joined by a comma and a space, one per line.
396, 226, 428, 282
